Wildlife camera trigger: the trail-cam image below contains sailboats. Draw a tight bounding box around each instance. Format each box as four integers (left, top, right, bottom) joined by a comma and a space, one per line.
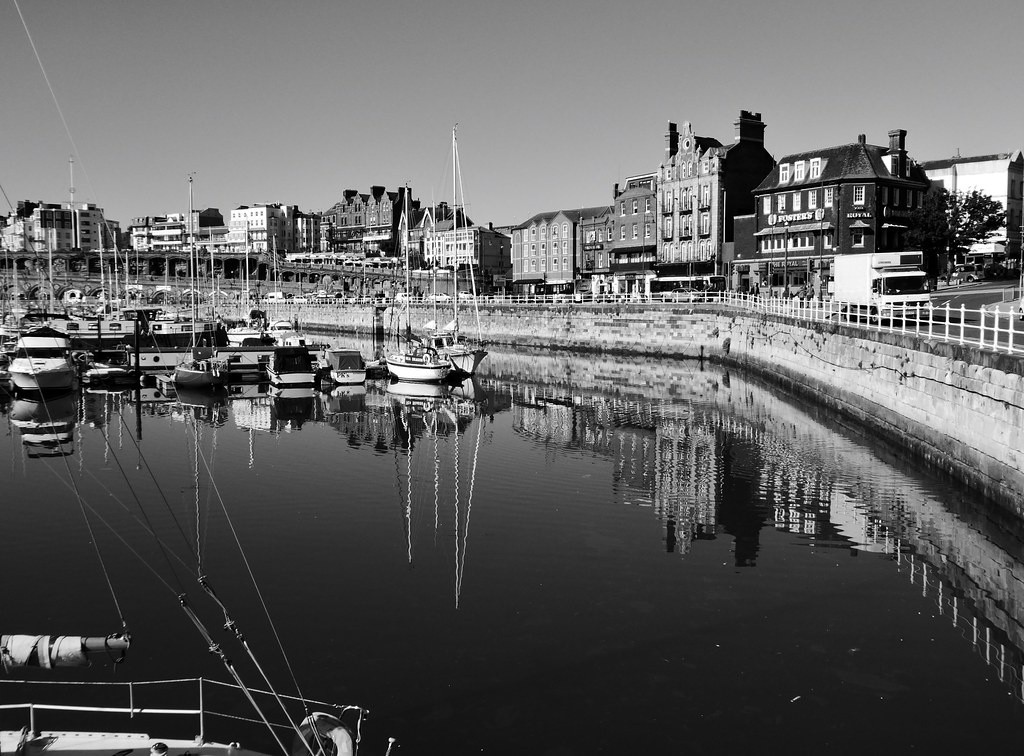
0, 0, 405, 756
0, 114, 491, 393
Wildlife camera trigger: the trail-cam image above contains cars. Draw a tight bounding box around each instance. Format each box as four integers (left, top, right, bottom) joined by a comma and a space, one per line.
671, 288, 702, 302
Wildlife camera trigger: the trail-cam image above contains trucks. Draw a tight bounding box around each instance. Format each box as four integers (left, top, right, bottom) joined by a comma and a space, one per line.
832, 250, 932, 324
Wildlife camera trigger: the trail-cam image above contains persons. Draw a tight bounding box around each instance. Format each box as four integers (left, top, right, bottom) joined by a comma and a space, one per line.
344, 275, 422, 303
783, 282, 814, 308
749, 282, 760, 302
702, 280, 711, 302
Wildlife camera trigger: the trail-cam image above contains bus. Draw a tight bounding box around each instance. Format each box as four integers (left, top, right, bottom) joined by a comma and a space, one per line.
651, 274, 728, 302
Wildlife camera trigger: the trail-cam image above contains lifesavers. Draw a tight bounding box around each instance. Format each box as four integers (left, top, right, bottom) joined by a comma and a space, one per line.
423, 401, 431, 412
423, 353, 431, 365
293, 714, 357, 755
